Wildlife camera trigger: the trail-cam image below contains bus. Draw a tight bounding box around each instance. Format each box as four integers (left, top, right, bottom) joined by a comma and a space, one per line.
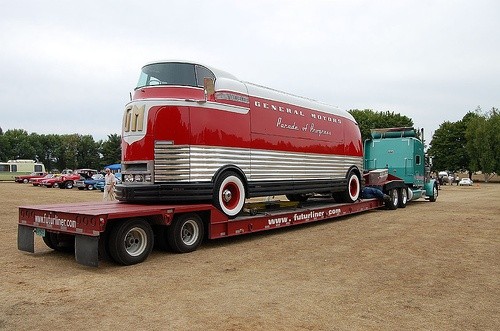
0, 160, 45, 181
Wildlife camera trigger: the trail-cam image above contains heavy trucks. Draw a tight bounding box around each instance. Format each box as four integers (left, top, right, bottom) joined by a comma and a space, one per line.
16, 126, 440, 271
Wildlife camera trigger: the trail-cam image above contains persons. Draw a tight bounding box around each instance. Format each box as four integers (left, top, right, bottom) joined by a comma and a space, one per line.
455, 175, 459, 186
104, 168, 115, 201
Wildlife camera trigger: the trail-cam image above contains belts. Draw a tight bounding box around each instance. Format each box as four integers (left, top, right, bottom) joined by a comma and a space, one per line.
107, 184, 111, 185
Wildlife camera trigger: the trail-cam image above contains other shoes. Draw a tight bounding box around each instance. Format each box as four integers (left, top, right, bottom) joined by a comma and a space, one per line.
383, 194, 394, 204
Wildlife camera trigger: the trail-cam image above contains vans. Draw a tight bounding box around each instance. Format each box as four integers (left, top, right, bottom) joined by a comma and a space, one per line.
111, 60, 364, 220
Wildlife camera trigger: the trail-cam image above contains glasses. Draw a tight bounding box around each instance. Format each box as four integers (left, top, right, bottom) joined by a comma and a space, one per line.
106, 171, 109, 172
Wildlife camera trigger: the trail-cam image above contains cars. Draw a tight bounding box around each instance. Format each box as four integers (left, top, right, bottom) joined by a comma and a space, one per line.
459, 177, 473, 187
14, 169, 121, 193
